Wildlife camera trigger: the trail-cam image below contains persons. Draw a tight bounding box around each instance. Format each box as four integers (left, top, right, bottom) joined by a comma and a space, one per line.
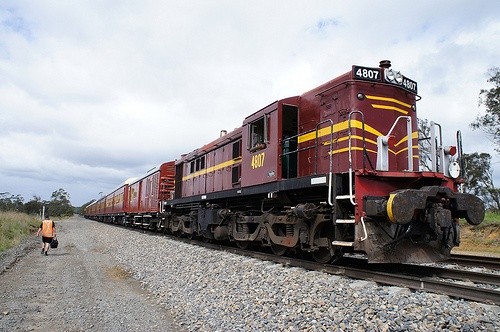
37, 214, 57, 256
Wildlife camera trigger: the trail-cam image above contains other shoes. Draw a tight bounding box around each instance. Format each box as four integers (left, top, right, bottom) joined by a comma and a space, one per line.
45, 251, 47, 255
41, 248, 44, 253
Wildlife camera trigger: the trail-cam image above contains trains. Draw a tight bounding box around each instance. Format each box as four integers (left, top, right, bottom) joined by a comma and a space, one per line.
82, 61, 486, 274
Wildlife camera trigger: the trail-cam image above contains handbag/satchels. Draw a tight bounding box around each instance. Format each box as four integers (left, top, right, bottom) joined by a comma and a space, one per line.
51, 234, 58, 248
